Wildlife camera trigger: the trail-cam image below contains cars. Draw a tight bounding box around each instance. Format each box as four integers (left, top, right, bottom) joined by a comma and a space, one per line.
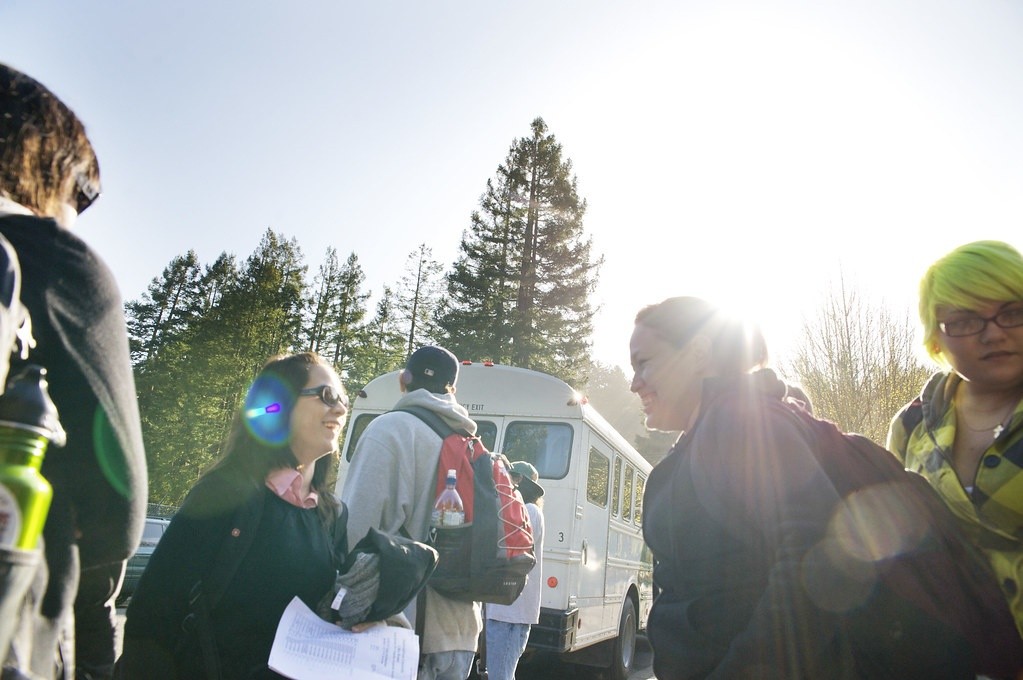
115, 515, 172, 606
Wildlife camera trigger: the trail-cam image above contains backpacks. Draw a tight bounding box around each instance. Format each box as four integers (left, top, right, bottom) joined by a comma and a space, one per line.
392, 406, 536, 605
699, 376, 1023, 680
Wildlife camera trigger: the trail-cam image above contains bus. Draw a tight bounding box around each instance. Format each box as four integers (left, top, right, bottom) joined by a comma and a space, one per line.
334, 360, 657, 680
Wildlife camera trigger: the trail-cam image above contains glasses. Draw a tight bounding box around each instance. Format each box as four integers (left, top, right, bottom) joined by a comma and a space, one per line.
74, 184, 99, 214
936, 302, 1023, 339
299, 385, 349, 409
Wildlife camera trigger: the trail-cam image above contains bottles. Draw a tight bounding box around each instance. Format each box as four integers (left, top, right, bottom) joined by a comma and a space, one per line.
432, 469, 465, 527
0, 362, 66, 554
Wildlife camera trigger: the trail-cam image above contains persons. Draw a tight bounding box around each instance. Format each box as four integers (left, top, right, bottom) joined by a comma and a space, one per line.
339, 347, 482, 680
113, 353, 442, 679
484, 461, 545, 680
0, 61, 148, 679
629, 298, 1023, 680
885, 240, 1022, 639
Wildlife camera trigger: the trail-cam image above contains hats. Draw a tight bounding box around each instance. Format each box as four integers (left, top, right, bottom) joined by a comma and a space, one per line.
510, 458, 545, 498
405, 345, 459, 388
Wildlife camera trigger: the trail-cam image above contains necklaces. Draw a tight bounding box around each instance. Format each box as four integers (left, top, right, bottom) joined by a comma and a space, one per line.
958, 397, 1018, 439
